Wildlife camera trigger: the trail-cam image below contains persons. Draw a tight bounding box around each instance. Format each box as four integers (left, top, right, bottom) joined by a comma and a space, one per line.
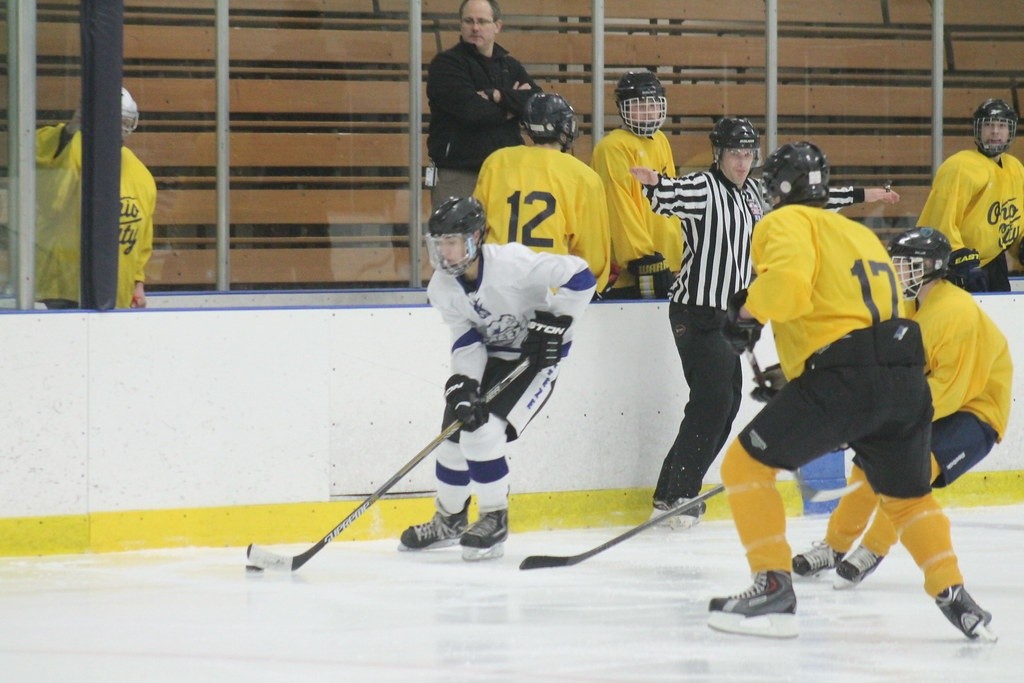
398, 198, 597, 561
33, 87, 156, 309
629, 114, 900, 532
708, 141, 1001, 645
588, 72, 683, 295
789, 226, 1013, 590
426, 0, 541, 216
474, 92, 611, 302
916, 97, 1024, 294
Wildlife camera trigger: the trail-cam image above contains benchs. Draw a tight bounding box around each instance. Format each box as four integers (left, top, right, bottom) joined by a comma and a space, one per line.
0, 0, 1024, 282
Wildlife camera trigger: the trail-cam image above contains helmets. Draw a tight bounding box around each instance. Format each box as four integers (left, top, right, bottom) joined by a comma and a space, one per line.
707, 116, 764, 171
76, 85, 139, 143
613, 69, 667, 137
428, 196, 487, 240
763, 141, 832, 209
974, 99, 1019, 158
519, 94, 578, 147
886, 226, 952, 300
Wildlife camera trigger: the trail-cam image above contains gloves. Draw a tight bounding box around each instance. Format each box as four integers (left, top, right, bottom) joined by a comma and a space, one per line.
718, 288, 763, 356
750, 363, 790, 403
519, 310, 574, 369
445, 373, 490, 432
625, 250, 677, 300
948, 247, 990, 293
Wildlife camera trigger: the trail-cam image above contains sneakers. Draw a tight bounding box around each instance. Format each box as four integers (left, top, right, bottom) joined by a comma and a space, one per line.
832, 541, 883, 590
397, 495, 471, 552
651, 495, 707, 533
793, 538, 847, 580
706, 569, 799, 641
935, 584, 997, 645
458, 508, 509, 561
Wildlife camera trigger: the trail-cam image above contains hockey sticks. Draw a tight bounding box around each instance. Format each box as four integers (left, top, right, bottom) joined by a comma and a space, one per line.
740, 329, 764, 386
244, 355, 529, 572
519, 484, 725, 571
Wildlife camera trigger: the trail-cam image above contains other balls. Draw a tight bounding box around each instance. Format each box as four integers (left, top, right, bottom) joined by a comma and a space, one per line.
246, 565, 264, 573
